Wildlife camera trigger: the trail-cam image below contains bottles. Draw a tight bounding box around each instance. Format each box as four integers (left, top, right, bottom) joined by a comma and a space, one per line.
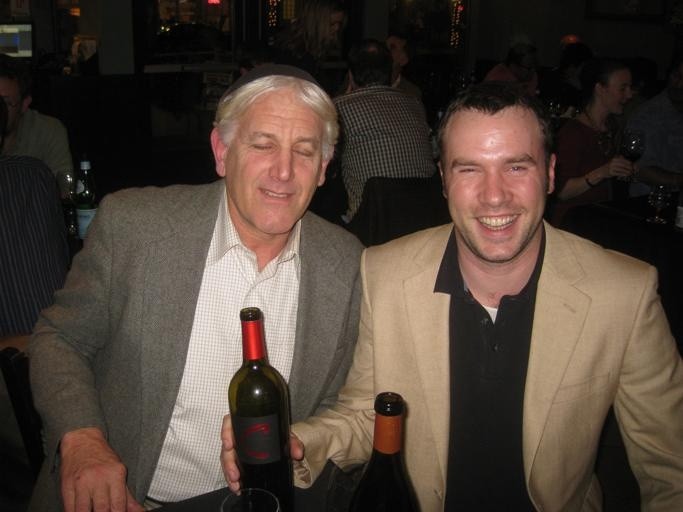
347, 391, 421, 512
75, 150, 98, 246
226, 307, 293, 512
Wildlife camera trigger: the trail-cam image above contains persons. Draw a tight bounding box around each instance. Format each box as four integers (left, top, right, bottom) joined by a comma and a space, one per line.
221, 77, 681, 512
11, 75, 370, 512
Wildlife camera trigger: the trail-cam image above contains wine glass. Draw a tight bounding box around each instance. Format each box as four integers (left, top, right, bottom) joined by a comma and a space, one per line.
617, 127, 645, 182
646, 184, 669, 225
220, 487, 281, 512
56, 170, 74, 234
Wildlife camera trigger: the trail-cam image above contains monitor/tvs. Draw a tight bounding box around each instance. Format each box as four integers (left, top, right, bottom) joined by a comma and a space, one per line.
0, 20, 37, 62
